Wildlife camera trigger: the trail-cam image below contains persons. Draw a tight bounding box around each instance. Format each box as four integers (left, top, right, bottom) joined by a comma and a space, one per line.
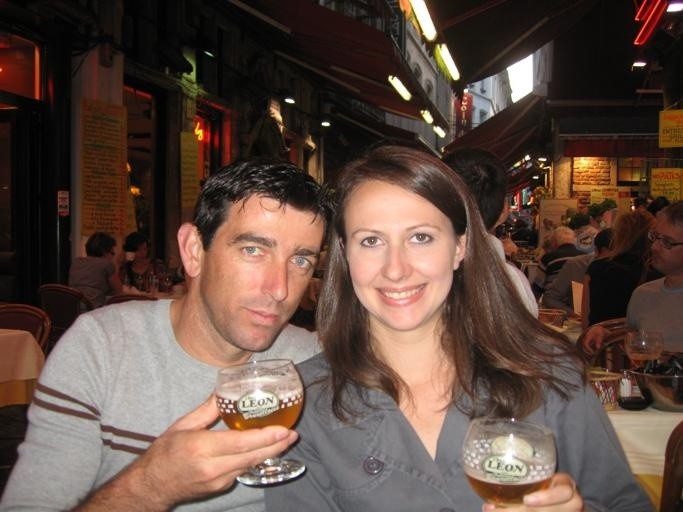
114, 233, 157, 292
1, 158, 325, 512
265, 145, 660, 512
441, 149, 538, 333
66, 230, 122, 309
507, 196, 683, 403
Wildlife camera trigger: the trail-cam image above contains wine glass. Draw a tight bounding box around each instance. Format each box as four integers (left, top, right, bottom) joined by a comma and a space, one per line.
622, 330, 665, 368
121, 270, 174, 296
213, 357, 308, 489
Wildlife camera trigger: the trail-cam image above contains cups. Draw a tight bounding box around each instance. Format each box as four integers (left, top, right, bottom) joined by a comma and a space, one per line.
460, 416, 561, 512
125, 252, 136, 262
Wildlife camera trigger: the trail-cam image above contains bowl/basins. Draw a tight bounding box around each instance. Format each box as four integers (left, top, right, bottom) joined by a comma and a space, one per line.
625, 359, 683, 413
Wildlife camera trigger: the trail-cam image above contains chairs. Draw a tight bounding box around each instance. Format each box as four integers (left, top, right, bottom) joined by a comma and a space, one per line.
514, 274, 682, 511
1, 269, 200, 488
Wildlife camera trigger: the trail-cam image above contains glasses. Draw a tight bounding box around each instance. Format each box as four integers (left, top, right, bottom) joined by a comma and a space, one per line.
646, 229, 683, 251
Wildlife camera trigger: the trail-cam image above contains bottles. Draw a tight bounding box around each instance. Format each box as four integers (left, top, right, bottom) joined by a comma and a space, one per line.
515, 246, 536, 261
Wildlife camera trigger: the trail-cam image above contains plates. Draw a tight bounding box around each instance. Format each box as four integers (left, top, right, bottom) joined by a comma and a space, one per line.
619, 393, 648, 412
